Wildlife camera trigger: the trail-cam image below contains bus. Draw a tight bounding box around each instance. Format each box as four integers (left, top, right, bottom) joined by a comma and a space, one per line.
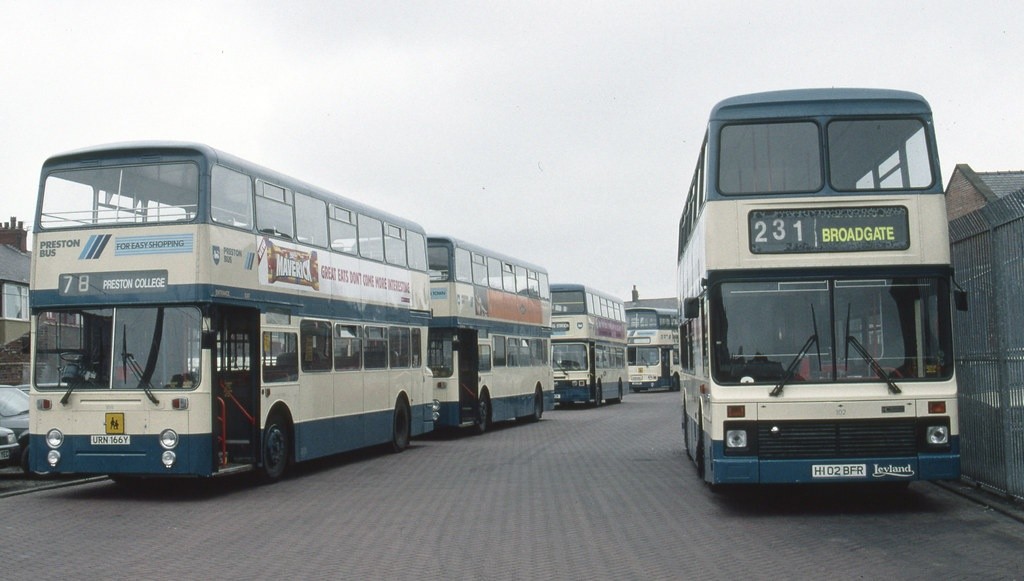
26, 138, 435, 486
625, 306, 680, 393
548, 282, 631, 408
425, 234, 555, 434
675, 84, 971, 488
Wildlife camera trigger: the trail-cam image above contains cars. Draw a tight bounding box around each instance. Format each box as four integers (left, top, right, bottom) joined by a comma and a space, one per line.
0, 426, 21, 470
0, 384, 61, 480
